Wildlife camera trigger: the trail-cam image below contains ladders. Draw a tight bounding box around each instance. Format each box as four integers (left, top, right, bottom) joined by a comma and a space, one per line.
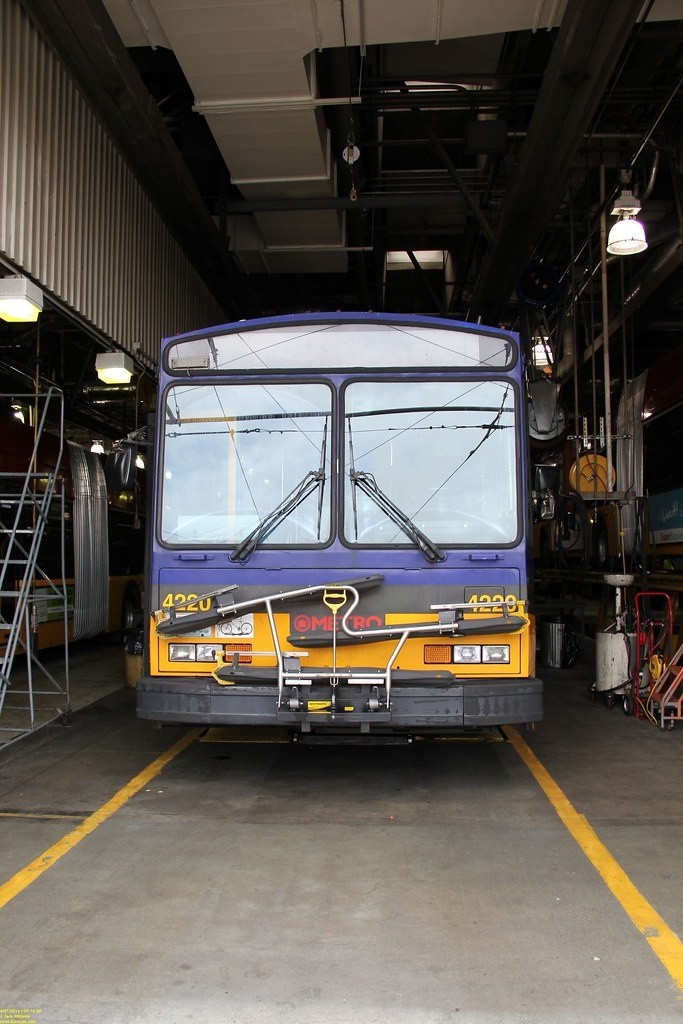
0, 385, 71, 754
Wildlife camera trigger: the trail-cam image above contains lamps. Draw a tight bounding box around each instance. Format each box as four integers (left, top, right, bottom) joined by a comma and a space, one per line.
11, 400, 24, 424
136, 455, 145, 469
532, 325, 553, 366
607, 170, 648, 256
0, 273, 43, 322
95, 349, 133, 384
91, 440, 105, 454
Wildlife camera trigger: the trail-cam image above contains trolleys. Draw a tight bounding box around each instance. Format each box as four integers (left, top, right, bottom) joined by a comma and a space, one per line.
621, 592, 678, 721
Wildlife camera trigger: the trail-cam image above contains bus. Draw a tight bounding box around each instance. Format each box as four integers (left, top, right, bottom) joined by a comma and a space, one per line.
0, 414, 146, 658
104, 312, 564, 751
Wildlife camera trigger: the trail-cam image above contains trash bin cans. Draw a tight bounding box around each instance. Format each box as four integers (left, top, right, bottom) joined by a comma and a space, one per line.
541, 612, 578, 669
123, 607, 145, 690
